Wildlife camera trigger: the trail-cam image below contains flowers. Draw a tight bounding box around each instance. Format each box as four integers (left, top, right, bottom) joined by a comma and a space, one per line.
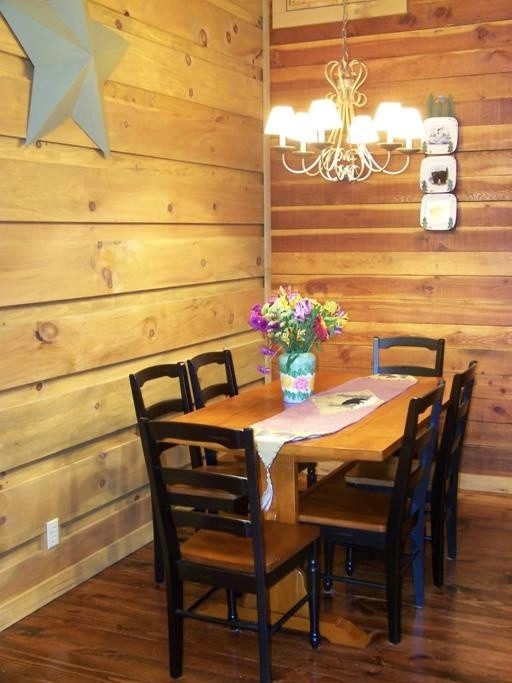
247, 283, 350, 375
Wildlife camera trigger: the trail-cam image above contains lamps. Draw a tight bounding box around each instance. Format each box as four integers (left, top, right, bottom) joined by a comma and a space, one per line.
263, 0, 424, 184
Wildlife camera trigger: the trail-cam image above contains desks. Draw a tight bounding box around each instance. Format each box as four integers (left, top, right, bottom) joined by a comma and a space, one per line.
151, 371, 446, 649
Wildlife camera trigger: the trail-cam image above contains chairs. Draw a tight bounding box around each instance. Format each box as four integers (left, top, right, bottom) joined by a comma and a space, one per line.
345, 358, 478, 591
187, 349, 318, 488
128, 361, 265, 585
297, 376, 448, 644
347, 334, 447, 486
135, 416, 323, 680
